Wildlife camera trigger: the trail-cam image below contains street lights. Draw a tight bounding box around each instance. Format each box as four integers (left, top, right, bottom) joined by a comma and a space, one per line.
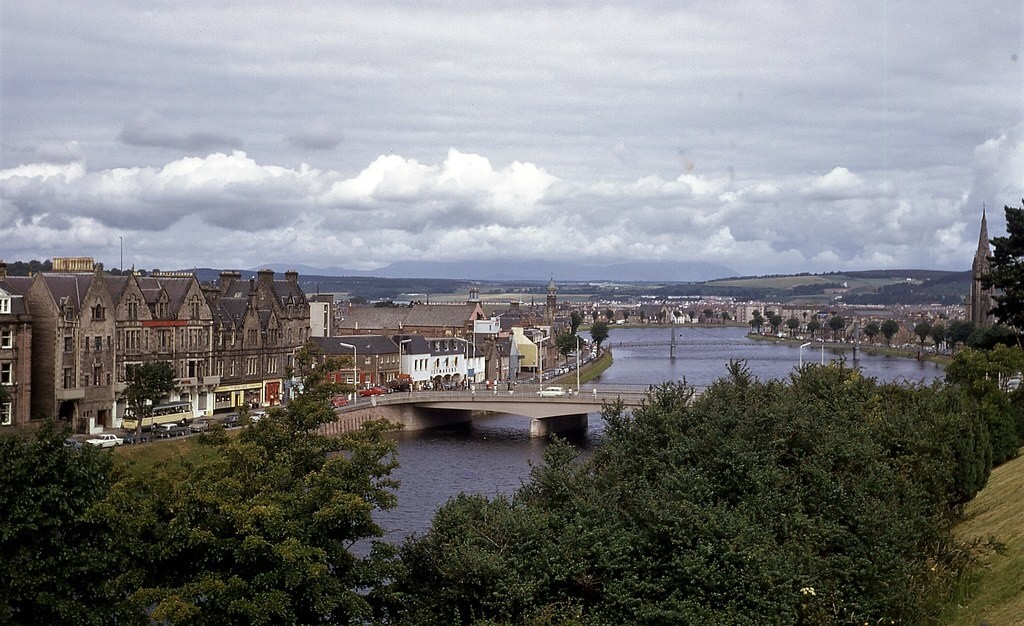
339, 342, 357, 405
800, 342, 813, 370
456, 337, 469, 389
292, 346, 304, 399
399, 339, 412, 376
539, 335, 550, 397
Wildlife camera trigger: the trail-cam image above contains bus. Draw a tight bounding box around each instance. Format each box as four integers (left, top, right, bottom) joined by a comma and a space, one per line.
122, 401, 194, 430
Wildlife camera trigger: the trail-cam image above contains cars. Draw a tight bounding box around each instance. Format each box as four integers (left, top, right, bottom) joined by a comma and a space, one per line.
85, 433, 124, 448
249, 411, 269, 423
815, 337, 938, 353
424, 380, 466, 389
512, 378, 539, 384
757, 327, 809, 340
358, 374, 414, 398
606, 318, 659, 324
331, 395, 348, 407
539, 339, 599, 384
123, 434, 147, 444
63, 439, 83, 452
189, 418, 211, 433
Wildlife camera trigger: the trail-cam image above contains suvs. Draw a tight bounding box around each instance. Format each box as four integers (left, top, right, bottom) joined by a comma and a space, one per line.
221, 414, 241, 428
536, 386, 566, 396
160, 423, 183, 433
